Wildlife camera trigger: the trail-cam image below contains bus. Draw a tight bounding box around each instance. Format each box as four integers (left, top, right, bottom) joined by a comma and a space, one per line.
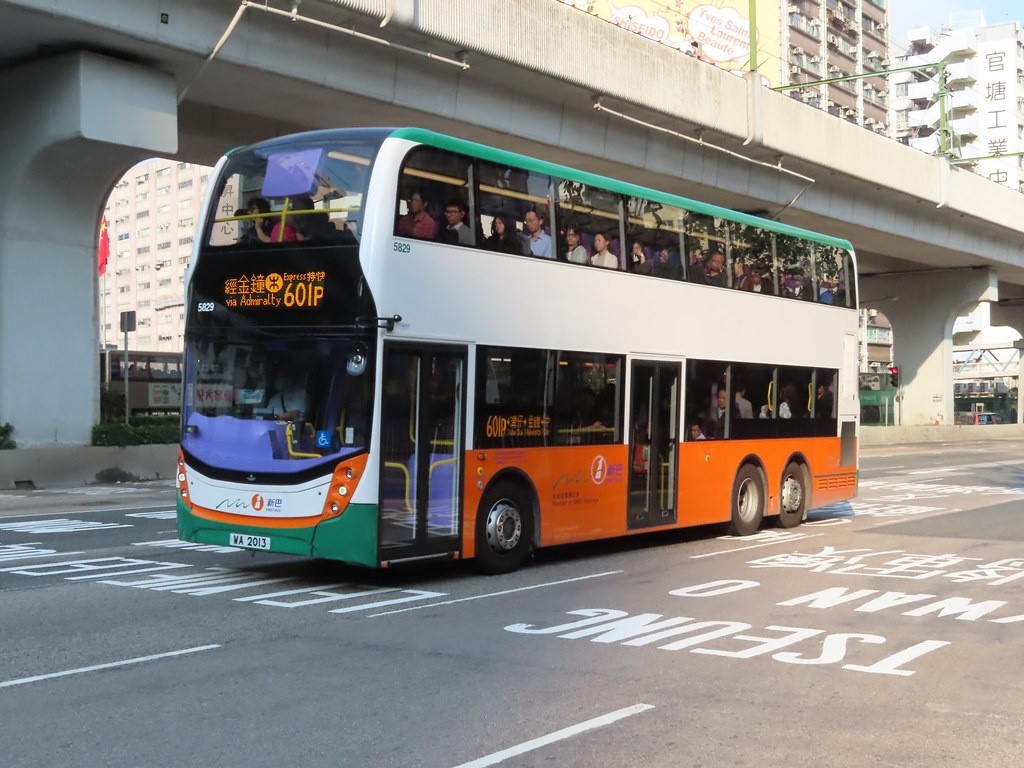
174, 126, 860, 575
100, 350, 185, 416
858, 372, 894, 426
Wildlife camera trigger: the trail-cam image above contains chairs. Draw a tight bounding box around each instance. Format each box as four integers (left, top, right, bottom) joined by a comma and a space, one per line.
522, 224, 622, 272
643, 245, 845, 290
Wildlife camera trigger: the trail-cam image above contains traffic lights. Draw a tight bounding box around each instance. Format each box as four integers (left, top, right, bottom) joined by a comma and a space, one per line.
889, 366, 898, 387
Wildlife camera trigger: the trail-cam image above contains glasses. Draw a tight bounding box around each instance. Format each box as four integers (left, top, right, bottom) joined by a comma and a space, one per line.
444, 210, 463, 215
524, 218, 540, 224
248, 207, 260, 210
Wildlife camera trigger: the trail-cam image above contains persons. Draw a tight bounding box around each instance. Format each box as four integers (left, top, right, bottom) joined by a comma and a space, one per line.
234, 185, 846, 307
246, 352, 834, 448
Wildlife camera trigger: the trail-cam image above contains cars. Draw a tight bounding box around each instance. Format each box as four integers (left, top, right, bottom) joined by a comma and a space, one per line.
973, 415, 994, 424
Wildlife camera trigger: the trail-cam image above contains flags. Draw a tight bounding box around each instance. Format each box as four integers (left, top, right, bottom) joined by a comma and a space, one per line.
97, 211, 110, 277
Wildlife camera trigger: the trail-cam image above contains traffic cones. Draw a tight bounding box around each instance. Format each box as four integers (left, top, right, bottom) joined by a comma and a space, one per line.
935, 413, 940, 427
974, 413, 979, 425
